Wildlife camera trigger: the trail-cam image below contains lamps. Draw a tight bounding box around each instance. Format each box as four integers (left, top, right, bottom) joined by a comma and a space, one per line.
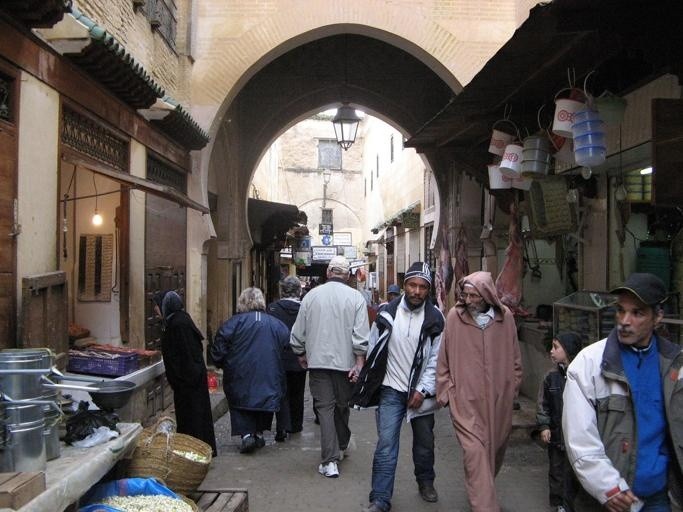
331, 101, 362, 151
615, 120, 629, 202
564, 164, 594, 206
91, 172, 104, 226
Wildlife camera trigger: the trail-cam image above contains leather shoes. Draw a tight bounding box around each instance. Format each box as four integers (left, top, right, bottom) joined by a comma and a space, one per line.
274, 427, 290, 442
415, 477, 441, 503
360, 500, 392, 512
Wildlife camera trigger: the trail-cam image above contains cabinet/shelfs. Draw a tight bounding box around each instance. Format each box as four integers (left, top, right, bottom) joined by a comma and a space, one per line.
187, 487, 249, 512
0, 423, 143, 512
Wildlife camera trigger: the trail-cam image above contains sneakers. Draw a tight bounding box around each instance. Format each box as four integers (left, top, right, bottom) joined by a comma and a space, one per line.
238, 434, 266, 456
316, 460, 341, 478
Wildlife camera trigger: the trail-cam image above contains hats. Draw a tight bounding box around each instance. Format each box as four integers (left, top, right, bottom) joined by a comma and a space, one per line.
385, 283, 404, 294
327, 254, 353, 273
609, 270, 669, 309
403, 258, 433, 286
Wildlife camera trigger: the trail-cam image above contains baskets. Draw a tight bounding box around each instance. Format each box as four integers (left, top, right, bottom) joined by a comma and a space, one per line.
64, 349, 138, 377
130, 414, 213, 497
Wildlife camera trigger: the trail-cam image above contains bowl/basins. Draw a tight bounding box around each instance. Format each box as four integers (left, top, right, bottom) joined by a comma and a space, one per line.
86, 380, 136, 408
520, 134, 551, 176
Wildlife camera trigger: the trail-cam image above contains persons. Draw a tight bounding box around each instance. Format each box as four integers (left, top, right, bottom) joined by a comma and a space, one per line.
267, 275, 306, 442
435, 271, 522, 512
154, 291, 217, 458
537, 332, 582, 512
348, 262, 447, 512
561, 274, 683, 512
210, 288, 291, 453
290, 255, 370, 477
276, 274, 401, 313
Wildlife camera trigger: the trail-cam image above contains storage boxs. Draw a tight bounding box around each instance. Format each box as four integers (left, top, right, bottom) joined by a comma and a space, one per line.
0, 470, 47, 511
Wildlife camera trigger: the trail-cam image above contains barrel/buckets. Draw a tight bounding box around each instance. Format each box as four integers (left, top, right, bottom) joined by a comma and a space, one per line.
0, 346, 104, 474
635, 240, 670, 288
485, 87, 588, 192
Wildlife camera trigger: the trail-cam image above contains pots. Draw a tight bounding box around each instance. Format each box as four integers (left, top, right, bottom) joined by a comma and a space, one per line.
0, 348, 76, 473
